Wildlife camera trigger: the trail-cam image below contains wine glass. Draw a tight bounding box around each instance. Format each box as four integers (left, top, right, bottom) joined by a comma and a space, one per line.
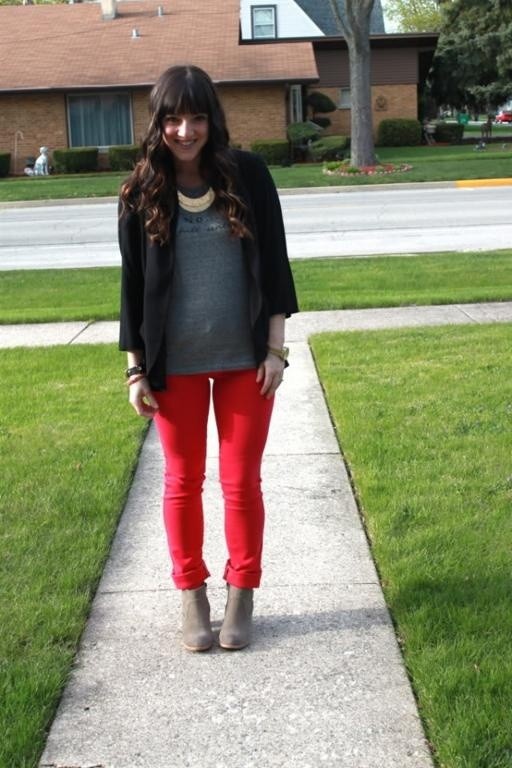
278, 377, 283, 385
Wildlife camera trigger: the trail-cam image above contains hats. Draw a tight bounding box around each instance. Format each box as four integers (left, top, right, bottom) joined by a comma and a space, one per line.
495, 110, 512, 124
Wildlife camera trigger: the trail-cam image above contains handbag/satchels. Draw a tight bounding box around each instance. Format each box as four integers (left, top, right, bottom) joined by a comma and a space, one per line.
125, 360, 145, 377
262, 341, 290, 364
128, 372, 147, 386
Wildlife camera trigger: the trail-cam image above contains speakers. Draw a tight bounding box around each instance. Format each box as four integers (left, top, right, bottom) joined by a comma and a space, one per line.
181, 582, 213, 651
219, 582, 254, 649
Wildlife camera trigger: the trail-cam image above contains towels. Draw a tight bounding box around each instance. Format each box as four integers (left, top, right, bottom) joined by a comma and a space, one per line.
458, 114, 466, 122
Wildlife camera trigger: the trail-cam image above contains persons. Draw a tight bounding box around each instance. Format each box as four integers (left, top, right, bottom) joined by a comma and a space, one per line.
113, 65, 301, 654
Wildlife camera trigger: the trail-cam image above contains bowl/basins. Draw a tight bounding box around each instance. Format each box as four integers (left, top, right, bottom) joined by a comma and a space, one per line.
175, 185, 217, 214
